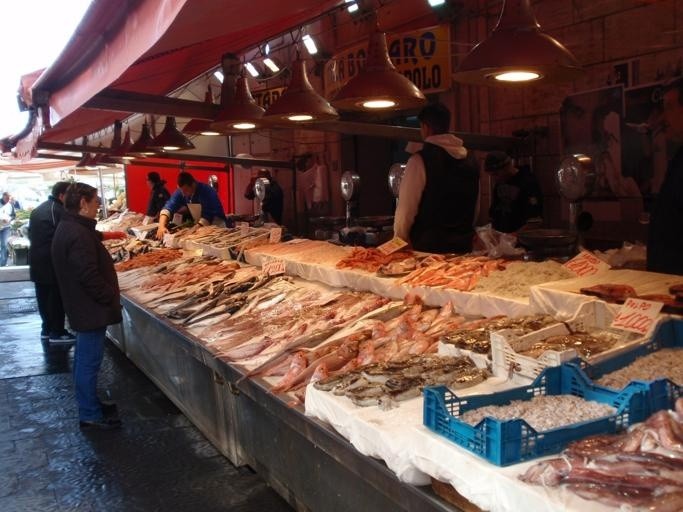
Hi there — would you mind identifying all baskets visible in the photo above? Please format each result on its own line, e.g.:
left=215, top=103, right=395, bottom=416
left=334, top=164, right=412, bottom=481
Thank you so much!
left=566, top=316, right=683, bottom=415
left=423, top=365, right=648, bottom=466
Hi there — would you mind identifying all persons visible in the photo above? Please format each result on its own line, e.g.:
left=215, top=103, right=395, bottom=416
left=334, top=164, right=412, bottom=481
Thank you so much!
left=482, top=151, right=544, bottom=245
left=27, top=180, right=128, bottom=345
left=155, top=170, right=228, bottom=243
left=393, top=101, right=483, bottom=258
left=567, top=148, right=645, bottom=259
left=51, top=181, right=125, bottom=431
left=573, top=104, right=619, bottom=162
left=244, top=167, right=286, bottom=228
left=645, top=77, right=681, bottom=276
left=9, top=197, right=23, bottom=211
left=0, top=189, right=19, bottom=267
left=141, top=170, right=172, bottom=226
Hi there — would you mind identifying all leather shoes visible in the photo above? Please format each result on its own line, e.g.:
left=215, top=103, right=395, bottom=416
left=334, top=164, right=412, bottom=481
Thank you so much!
left=98, top=400, right=117, bottom=413
left=79, top=416, right=120, bottom=431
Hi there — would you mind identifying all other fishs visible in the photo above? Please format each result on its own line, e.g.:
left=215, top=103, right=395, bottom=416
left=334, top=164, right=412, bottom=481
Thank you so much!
left=204, top=291, right=506, bottom=406
left=141, top=255, right=289, bottom=328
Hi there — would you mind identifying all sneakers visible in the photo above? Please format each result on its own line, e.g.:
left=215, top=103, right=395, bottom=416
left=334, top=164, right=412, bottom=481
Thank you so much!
left=41, top=330, right=49, bottom=339
left=49, top=332, right=76, bottom=342
